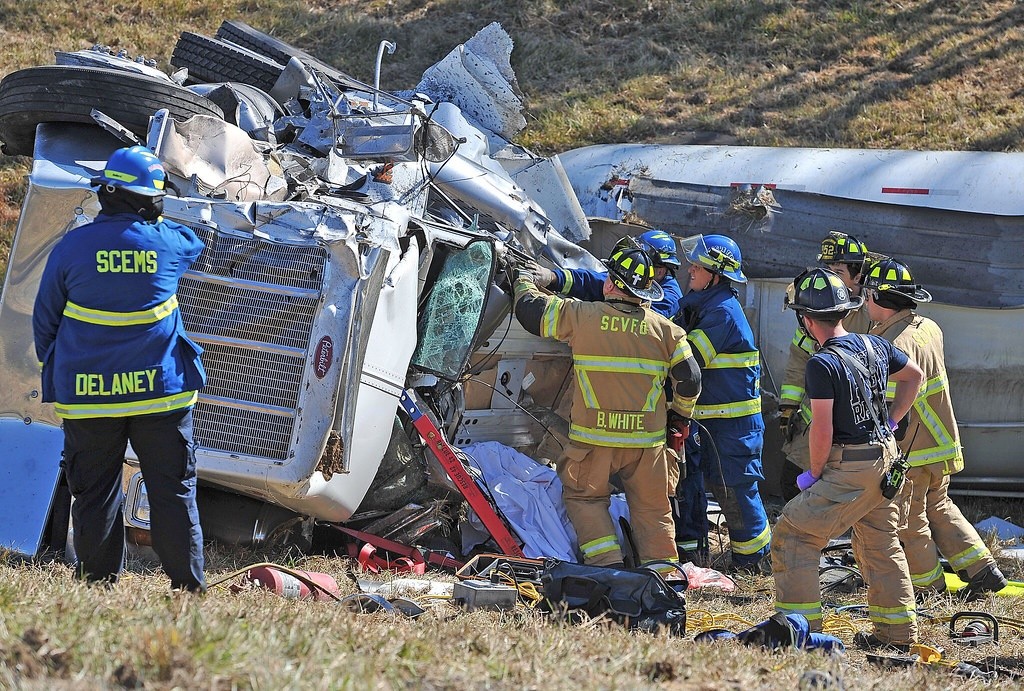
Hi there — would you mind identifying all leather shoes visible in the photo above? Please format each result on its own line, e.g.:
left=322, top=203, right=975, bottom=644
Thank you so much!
left=852, top=629, right=909, bottom=654
left=956, top=567, right=1008, bottom=602
left=914, top=584, right=948, bottom=605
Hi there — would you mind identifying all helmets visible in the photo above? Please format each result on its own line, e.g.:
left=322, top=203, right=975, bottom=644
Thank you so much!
left=687, top=235, right=748, bottom=284
left=857, top=259, right=933, bottom=303
left=94, top=145, right=167, bottom=197
left=635, top=229, right=681, bottom=265
left=787, top=267, right=864, bottom=314
left=820, top=232, right=868, bottom=263
left=603, top=245, right=665, bottom=302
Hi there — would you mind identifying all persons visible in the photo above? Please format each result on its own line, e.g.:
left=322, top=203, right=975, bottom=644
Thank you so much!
left=33, top=146, right=206, bottom=593
left=525, top=231, right=710, bottom=569
left=515, top=246, right=702, bottom=583
left=771, top=267, right=923, bottom=652
left=776, top=231, right=879, bottom=501
left=861, top=258, right=1007, bottom=602
left=663, top=233, right=773, bottom=575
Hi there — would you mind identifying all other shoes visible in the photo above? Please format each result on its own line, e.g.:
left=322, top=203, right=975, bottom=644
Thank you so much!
left=725, top=561, right=759, bottom=574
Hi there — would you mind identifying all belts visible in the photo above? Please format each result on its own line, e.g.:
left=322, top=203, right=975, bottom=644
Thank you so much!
left=840, top=448, right=884, bottom=461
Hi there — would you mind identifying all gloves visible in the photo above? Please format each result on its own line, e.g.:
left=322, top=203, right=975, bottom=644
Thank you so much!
left=779, top=406, right=803, bottom=443
left=524, top=259, right=553, bottom=286
left=512, top=268, right=535, bottom=283
left=888, top=417, right=899, bottom=433
left=796, top=472, right=817, bottom=492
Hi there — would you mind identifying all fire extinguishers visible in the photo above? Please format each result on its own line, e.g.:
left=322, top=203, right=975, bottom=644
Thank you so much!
left=207, top=551, right=340, bottom=603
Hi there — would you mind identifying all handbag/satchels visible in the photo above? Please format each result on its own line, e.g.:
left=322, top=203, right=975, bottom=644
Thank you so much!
left=536, top=558, right=689, bottom=640
left=697, top=612, right=844, bottom=655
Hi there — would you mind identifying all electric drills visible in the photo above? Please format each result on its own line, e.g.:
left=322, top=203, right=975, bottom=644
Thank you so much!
left=667, top=416, right=692, bottom=483
